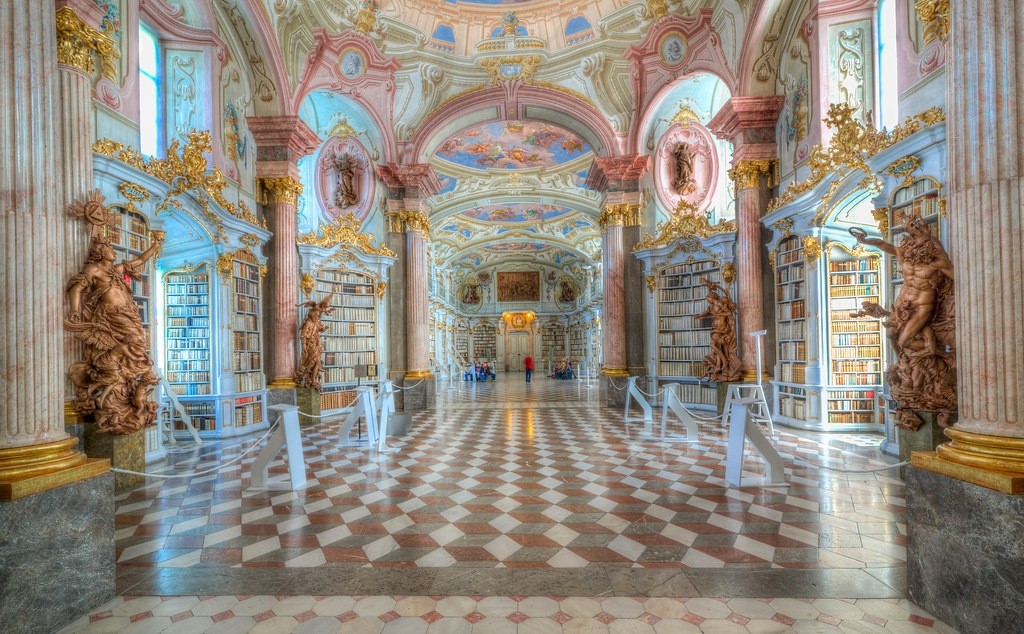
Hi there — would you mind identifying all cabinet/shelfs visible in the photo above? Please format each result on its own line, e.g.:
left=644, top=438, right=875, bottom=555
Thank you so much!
left=541, top=317, right=597, bottom=372
left=888, top=174, right=943, bottom=450
left=771, top=233, right=810, bottom=425
left=160, top=257, right=213, bottom=397
left=314, top=267, right=382, bottom=385
left=104, top=201, right=154, bottom=425
left=427, top=296, right=499, bottom=379
left=825, top=242, right=883, bottom=433
left=230, top=249, right=263, bottom=392
left=656, top=258, right=722, bottom=408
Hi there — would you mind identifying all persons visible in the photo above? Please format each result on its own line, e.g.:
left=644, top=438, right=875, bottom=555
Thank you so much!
left=465, top=360, right=497, bottom=382
left=524, top=355, right=534, bottom=382
left=333, top=159, right=354, bottom=197
left=857, top=216, right=954, bottom=389
left=294, top=289, right=336, bottom=391
left=694, top=278, right=742, bottom=382
left=553, top=358, right=578, bottom=378
left=68, top=237, right=160, bottom=419
left=674, top=142, right=694, bottom=182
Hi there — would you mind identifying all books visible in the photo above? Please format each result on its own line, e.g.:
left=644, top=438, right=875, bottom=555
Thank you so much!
left=657, top=261, right=721, bottom=405
left=304, top=270, right=377, bottom=411
left=776, top=177, right=939, bottom=424
left=102, top=211, right=262, bottom=430
left=429, top=318, right=587, bottom=370
